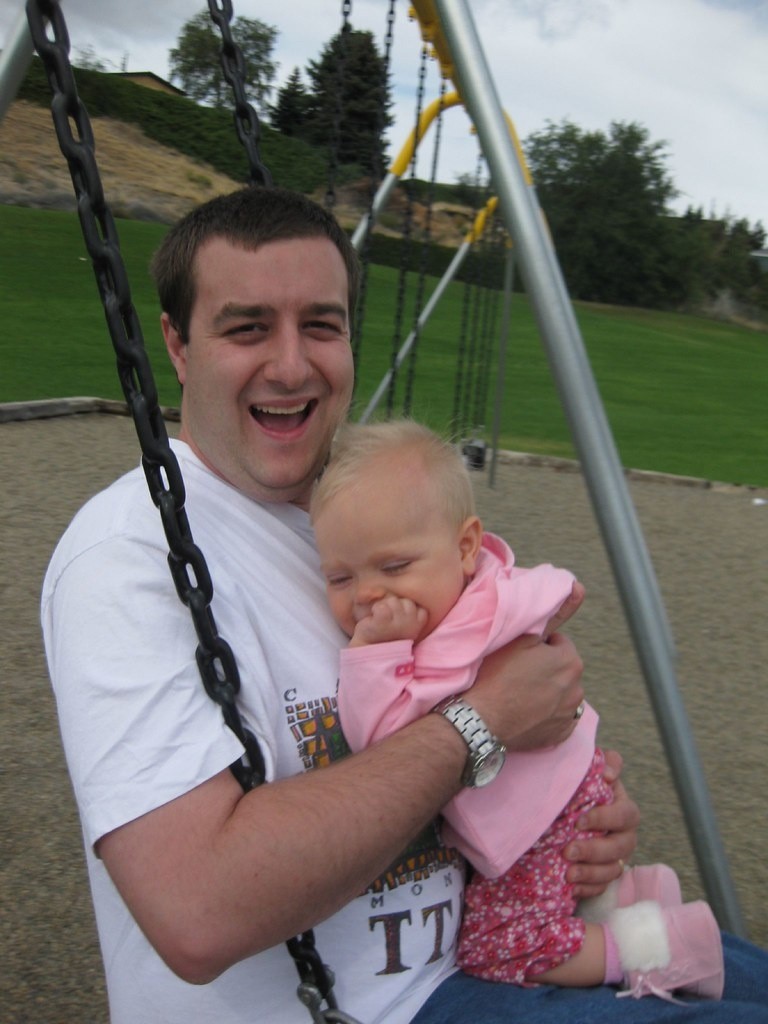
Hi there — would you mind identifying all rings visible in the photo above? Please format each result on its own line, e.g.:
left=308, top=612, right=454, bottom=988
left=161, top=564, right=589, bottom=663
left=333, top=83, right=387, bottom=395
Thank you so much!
left=575, top=705, right=585, bottom=718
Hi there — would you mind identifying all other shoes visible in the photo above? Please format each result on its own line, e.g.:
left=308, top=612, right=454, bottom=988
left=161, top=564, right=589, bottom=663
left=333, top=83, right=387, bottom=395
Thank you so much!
left=615, top=864, right=682, bottom=911
left=608, top=900, right=724, bottom=1006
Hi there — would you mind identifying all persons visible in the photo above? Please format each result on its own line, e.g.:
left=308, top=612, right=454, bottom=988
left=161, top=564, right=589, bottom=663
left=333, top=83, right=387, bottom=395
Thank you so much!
left=41, top=188, right=768, bottom=1024
left=307, top=419, right=723, bottom=997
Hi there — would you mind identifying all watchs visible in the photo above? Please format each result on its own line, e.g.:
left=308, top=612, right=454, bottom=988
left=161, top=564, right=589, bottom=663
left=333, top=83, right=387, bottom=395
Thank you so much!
left=432, top=694, right=506, bottom=787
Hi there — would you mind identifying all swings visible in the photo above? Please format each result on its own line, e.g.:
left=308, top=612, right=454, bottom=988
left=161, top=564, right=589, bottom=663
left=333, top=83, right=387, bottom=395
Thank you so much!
left=24, top=1, right=524, bottom=1024
